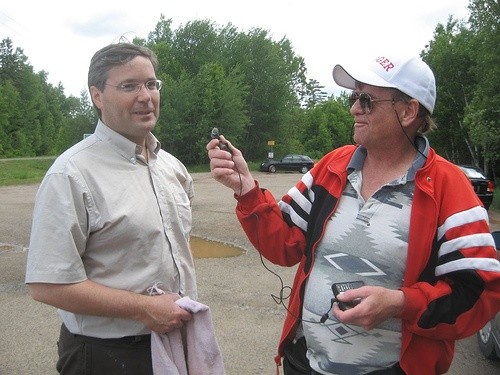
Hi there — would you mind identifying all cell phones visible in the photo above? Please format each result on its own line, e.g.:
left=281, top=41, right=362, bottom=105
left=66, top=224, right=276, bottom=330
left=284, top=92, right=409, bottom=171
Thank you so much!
left=332, top=281, right=365, bottom=311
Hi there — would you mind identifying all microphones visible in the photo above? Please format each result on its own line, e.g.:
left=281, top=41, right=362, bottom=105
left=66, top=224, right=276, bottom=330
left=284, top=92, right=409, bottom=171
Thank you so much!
left=211, top=128, right=227, bottom=151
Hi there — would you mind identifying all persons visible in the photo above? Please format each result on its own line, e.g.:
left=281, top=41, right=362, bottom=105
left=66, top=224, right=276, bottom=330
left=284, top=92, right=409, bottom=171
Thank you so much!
left=24, top=43, right=195, bottom=375
left=206, top=52, right=499, bottom=375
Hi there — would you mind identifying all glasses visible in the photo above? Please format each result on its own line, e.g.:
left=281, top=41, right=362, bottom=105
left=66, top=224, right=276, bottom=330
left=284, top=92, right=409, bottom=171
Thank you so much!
left=104, top=80, right=162, bottom=95
left=349, top=91, right=408, bottom=114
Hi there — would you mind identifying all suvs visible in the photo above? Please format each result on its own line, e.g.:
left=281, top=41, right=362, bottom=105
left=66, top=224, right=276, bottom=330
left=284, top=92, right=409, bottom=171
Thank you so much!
left=261, top=154, right=314, bottom=174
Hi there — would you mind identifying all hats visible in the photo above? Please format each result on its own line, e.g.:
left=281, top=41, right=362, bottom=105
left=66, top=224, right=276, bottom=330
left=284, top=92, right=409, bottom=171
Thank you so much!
left=332, top=55, right=436, bottom=115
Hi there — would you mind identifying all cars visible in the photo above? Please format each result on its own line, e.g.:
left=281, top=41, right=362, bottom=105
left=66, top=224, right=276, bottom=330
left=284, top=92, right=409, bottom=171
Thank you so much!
left=455, top=164, right=494, bottom=210
